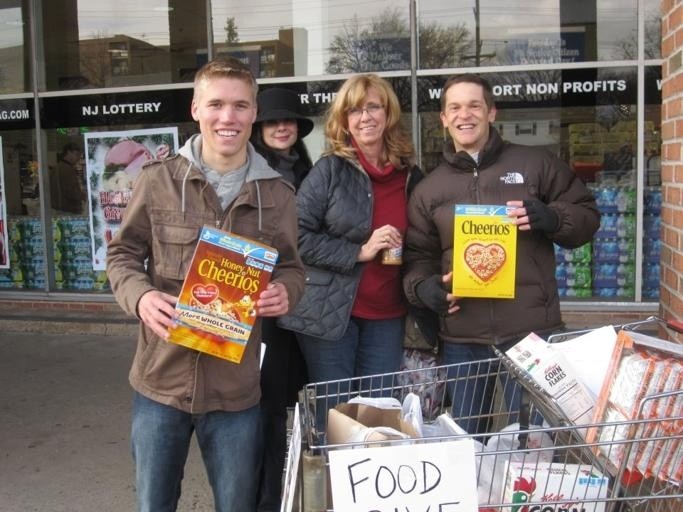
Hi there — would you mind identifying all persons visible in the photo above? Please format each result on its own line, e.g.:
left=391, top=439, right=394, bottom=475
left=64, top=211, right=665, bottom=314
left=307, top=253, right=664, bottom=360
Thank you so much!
left=276, top=68, right=424, bottom=416
left=397, top=74, right=601, bottom=446
left=102, top=54, right=306, bottom=512
left=245, top=87, right=315, bottom=480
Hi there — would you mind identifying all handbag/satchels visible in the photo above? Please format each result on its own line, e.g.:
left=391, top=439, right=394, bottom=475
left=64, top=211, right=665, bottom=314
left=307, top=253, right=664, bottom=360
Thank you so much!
left=396, top=308, right=448, bottom=421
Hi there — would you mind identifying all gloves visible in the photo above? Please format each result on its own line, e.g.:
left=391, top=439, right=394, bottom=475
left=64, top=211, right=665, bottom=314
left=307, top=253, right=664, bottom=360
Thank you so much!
left=415, top=273, right=450, bottom=318
left=522, top=198, right=559, bottom=232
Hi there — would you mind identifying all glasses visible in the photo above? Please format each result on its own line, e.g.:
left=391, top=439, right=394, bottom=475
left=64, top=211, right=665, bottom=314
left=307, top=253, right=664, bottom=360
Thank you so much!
left=347, top=103, right=387, bottom=115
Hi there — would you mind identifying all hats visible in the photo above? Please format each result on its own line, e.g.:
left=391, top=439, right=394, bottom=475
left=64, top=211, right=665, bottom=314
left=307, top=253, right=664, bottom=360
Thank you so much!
left=251, top=88, right=314, bottom=138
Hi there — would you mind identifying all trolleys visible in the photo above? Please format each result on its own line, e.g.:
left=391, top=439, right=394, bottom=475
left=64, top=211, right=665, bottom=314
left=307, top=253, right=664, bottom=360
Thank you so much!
left=280, top=315, right=683, bottom=512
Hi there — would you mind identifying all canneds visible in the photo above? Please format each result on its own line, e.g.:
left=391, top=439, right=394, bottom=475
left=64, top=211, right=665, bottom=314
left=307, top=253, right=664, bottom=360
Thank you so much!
left=381, top=234, right=403, bottom=265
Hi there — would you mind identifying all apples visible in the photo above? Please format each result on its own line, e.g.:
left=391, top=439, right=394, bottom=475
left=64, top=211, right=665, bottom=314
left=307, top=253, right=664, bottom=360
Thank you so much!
left=511, top=492, right=527, bottom=512
